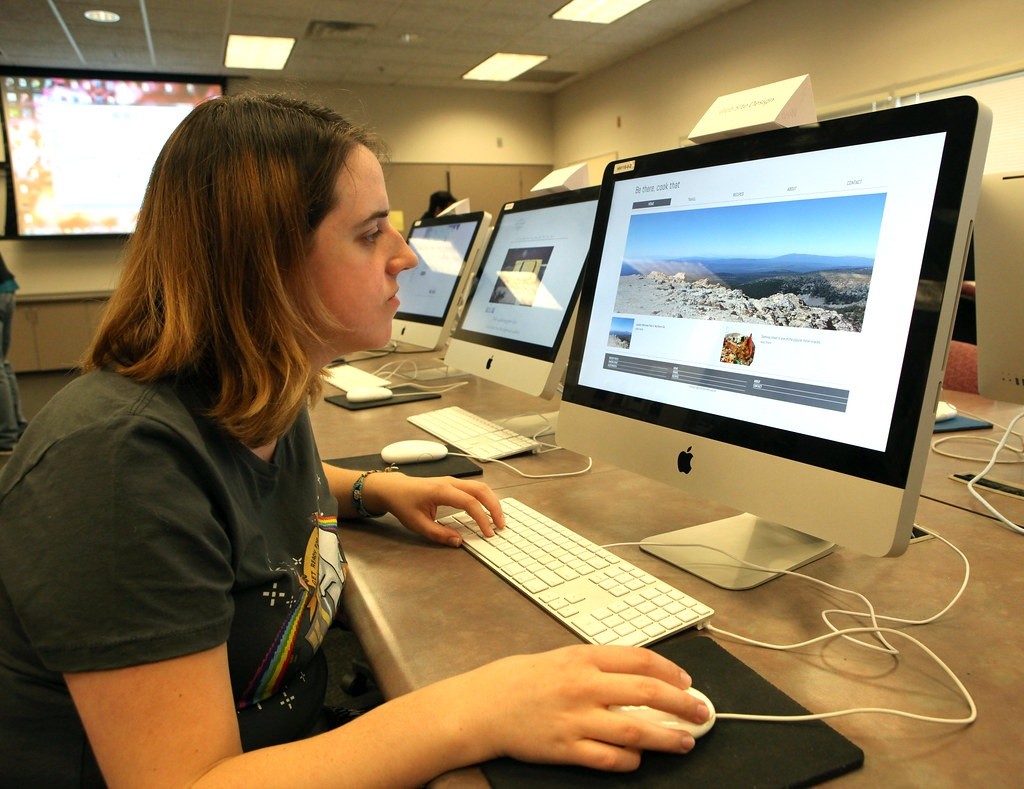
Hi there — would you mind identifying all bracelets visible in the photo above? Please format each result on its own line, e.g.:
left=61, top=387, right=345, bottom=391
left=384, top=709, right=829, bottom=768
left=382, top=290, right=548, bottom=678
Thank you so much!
left=353, top=463, right=399, bottom=518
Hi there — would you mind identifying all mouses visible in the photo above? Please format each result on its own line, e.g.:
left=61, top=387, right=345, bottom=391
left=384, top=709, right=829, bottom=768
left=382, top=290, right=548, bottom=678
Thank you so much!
left=381, top=440, right=448, bottom=464
left=346, top=388, right=393, bottom=402
left=935, top=400, right=958, bottom=422
left=607, top=685, right=716, bottom=738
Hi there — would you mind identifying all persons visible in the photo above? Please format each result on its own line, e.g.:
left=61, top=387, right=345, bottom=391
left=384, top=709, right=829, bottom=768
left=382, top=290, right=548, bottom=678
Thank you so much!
left=418, top=190, right=459, bottom=219
left=0, top=250, right=28, bottom=456
left=0, top=89, right=708, bottom=789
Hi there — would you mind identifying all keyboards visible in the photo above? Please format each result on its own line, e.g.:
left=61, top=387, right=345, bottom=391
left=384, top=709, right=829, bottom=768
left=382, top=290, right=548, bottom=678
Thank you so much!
left=430, top=497, right=714, bottom=649
left=318, top=364, right=392, bottom=392
left=407, top=406, right=541, bottom=462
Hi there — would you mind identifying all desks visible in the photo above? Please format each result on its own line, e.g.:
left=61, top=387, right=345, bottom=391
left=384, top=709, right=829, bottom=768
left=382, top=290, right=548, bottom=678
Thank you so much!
left=309, top=314, right=1024, bottom=789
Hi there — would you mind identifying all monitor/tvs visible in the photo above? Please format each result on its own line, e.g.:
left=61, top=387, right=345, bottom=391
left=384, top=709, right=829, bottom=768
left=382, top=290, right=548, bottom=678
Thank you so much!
left=394, top=94, right=1024, bottom=591
